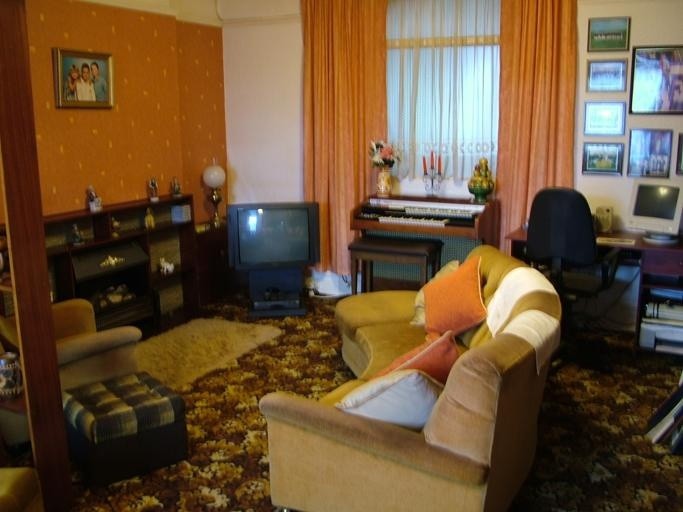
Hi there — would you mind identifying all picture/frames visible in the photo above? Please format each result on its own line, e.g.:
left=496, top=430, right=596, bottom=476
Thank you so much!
left=584, top=101, right=625, bottom=136
left=628, top=129, right=672, bottom=178
left=585, top=60, right=627, bottom=93
left=53, top=48, right=114, bottom=109
left=588, top=16, right=631, bottom=51
left=675, top=134, right=683, bottom=175
left=582, top=143, right=624, bottom=175
left=629, top=47, right=683, bottom=114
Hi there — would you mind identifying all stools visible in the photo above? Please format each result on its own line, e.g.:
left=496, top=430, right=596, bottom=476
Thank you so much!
left=62, top=371, right=188, bottom=487
left=348, top=236, right=445, bottom=295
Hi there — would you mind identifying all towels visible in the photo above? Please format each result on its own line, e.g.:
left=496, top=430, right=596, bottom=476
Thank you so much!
left=486, top=267, right=559, bottom=339
left=497, top=310, right=560, bottom=375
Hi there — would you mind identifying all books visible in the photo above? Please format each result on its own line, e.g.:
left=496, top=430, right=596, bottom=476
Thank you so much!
left=643, top=286, right=683, bottom=326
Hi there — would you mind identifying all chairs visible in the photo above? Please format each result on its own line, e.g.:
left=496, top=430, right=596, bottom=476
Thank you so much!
left=527, top=186, right=623, bottom=301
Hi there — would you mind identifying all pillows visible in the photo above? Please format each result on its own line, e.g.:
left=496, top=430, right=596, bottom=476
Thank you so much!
left=335, top=370, right=445, bottom=428
left=366, top=330, right=460, bottom=379
left=420, top=257, right=486, bottom=334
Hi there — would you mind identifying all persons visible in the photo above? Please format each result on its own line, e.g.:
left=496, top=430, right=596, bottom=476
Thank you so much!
left=89, top=63, right=107, bottom=100
left=64, top=64, right=81, bottom=101
left=75, top=63, right=96, bottom=101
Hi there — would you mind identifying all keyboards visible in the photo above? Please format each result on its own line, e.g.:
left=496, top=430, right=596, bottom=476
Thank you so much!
left=595, top=238, right=636, bottom=246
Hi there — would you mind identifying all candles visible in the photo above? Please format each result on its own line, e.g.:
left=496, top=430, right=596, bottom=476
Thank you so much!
left=422, top=155, right=427, bottom=175
left=430, top=150, right=434, bottom=169
left=438, top=155, right=442, bottom=175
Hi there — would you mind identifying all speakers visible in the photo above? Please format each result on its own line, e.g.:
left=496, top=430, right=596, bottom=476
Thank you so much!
left=595, top=206, right=612, bottom=234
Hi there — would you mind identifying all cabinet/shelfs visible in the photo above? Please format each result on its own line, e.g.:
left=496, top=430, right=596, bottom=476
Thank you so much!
left=0, top=191, right=204, bottom=339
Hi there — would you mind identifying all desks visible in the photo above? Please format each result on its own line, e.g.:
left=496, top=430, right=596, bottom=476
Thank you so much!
left=506, top=223, right=683, bottom=358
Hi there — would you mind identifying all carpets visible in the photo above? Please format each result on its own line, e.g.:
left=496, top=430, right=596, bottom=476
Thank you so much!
left=0, top=286, right=681, bottom=512
left=133, top=318, right=281, bottom=390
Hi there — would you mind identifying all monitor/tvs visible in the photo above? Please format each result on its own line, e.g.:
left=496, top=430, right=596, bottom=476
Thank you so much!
left=627, top=178, right=681, bottom=245
left=227, top=202, right=321, bottom=268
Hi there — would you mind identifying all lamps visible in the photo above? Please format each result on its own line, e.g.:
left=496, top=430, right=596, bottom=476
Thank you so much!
left=202, top=157, right=226, bottom=233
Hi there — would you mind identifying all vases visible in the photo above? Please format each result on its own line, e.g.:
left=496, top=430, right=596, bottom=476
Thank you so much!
left=376, top=167, right=391, bottom=198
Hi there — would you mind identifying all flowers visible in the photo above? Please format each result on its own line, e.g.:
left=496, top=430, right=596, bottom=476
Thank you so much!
left=367, top=138, right=401, bottom=167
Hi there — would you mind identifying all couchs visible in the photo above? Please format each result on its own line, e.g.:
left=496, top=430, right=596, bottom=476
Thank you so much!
left=0, top=298, right=142, bottom=454
left=259, top=245, right=561, bottom=512
